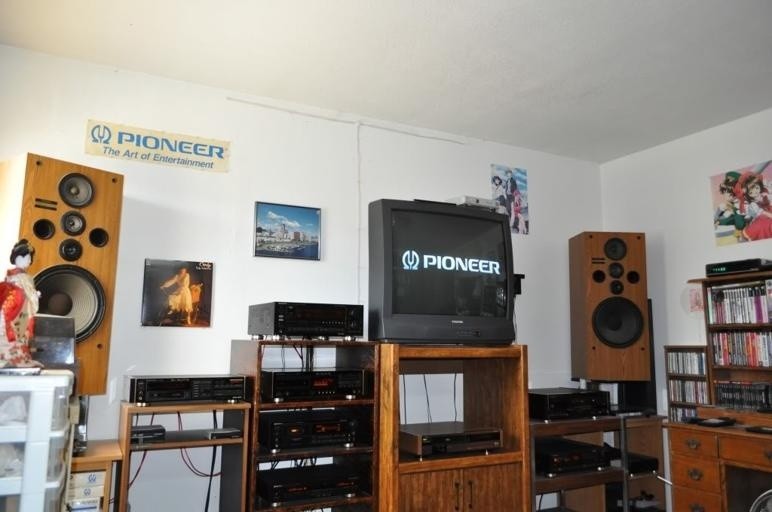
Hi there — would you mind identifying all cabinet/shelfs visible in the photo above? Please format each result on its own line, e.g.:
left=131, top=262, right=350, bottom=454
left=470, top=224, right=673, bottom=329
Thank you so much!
left=697, top=269, right=771, bottom=428
left=0, top=370, right=72, bottom=512
left=378, top=341, right=532, bottom=512
left=119, top=399, right=250, bottom=511
left=662, top=341, right=716, bottom=425
left=231, top=339, right=377, bottom=511
left=65, top=439, right=123, bottom=511
left=531, top=417, right=669, bottom=512
left=668, top=413, right=771, bottom=512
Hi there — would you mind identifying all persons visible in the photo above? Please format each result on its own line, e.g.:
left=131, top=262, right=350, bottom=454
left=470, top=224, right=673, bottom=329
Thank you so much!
left=734, top=171, right=772, bottom=239
left=159, top=267, right=193, bottom=325
left=492, top=175, right=507, bottom=207
left=0, top=237, right=45, bottom=368
left=509, top=190, right=528, bottom=234
left=714, top=171, right=753, bottom=239
left=495, top=195, right=509, bottom=216
left=504, top=170, right=517, bottom=212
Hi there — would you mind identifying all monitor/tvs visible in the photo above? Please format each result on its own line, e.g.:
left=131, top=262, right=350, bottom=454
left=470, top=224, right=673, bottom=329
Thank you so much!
left=368, top=199, right=517, bottom=346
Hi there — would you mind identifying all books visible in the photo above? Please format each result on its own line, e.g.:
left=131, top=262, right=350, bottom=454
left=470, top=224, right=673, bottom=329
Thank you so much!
left=706, top=279, right=772, bottom=325
left=668, top=379, right=710, bottom=404
left=666, top=352, right=706, bottom=376
left=710, top=331, right=772, bottom=367
left=670, top=407, right=695, bottom=422
left=713, top=379, right=771, bottom=412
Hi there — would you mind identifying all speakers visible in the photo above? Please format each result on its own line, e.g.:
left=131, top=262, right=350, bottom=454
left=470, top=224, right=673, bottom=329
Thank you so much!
left=18, top=151, right=124, bottom=397
left=568, top=231, right=651, bottom=382
